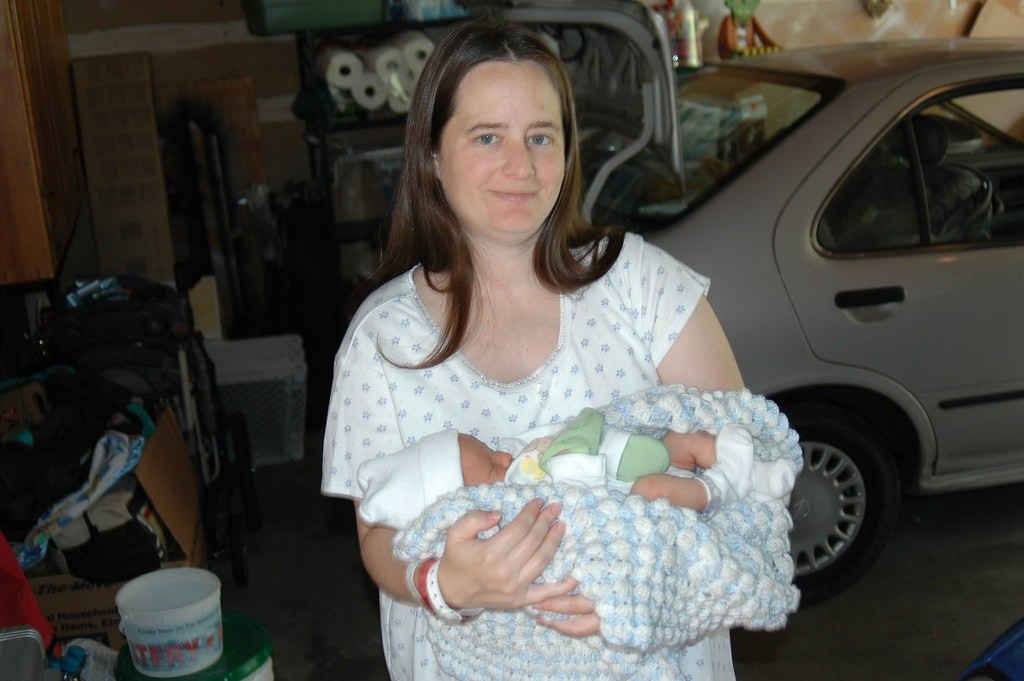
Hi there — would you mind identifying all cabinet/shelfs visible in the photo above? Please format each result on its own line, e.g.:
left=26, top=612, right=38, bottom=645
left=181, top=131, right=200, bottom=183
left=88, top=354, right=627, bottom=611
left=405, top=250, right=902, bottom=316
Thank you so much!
left=296, top=18, right=472, bottom=301
left=0, top=0, right=85, bottom=285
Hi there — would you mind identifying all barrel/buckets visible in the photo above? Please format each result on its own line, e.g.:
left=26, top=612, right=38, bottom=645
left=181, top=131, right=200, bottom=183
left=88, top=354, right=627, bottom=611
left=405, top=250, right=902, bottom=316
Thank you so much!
left=116, top=568, right=222, bottom=677
left=114, top=613, right=274, bottom=681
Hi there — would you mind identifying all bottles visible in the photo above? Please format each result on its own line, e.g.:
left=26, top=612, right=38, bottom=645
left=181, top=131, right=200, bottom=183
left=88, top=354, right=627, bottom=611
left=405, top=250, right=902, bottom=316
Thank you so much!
left=47, top=641, right=85, bottom=681
left=652, top=0, right=699, bottom=69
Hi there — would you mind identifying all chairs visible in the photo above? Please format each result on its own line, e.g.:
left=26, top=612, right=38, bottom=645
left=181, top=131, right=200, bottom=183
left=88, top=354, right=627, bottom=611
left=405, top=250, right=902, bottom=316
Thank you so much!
left=886, top=115, right=992, bottom=242
left=30, top=274, right=261, bottom=586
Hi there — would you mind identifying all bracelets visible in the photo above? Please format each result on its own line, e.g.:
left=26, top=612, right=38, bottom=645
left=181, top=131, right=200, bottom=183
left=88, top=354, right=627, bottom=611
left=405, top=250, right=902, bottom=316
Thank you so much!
left=406, top=557, right=485, bottom=624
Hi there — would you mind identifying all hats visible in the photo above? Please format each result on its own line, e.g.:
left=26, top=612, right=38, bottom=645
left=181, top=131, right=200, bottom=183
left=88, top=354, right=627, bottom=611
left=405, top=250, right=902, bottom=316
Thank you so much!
left=356, top=428, right=466, bottom=529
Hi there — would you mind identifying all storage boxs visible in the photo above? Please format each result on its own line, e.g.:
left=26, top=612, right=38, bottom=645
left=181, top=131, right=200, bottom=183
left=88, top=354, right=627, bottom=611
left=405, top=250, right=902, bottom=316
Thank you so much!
left=27, top=403, right=209, bottom=649
left=239, top=0, right=385, bottom=37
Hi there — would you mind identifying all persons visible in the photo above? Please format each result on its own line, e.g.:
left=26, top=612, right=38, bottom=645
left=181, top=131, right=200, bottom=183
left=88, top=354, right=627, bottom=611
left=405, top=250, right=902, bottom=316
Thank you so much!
left=357, top=408, right=797, bottom=529
left=322, top=19, right=746, bottom=681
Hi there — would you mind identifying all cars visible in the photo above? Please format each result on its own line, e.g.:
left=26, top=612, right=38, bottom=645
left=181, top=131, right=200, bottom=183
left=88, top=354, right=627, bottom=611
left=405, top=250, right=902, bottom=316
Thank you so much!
left=445, top=0, right=1024, bottom=610
